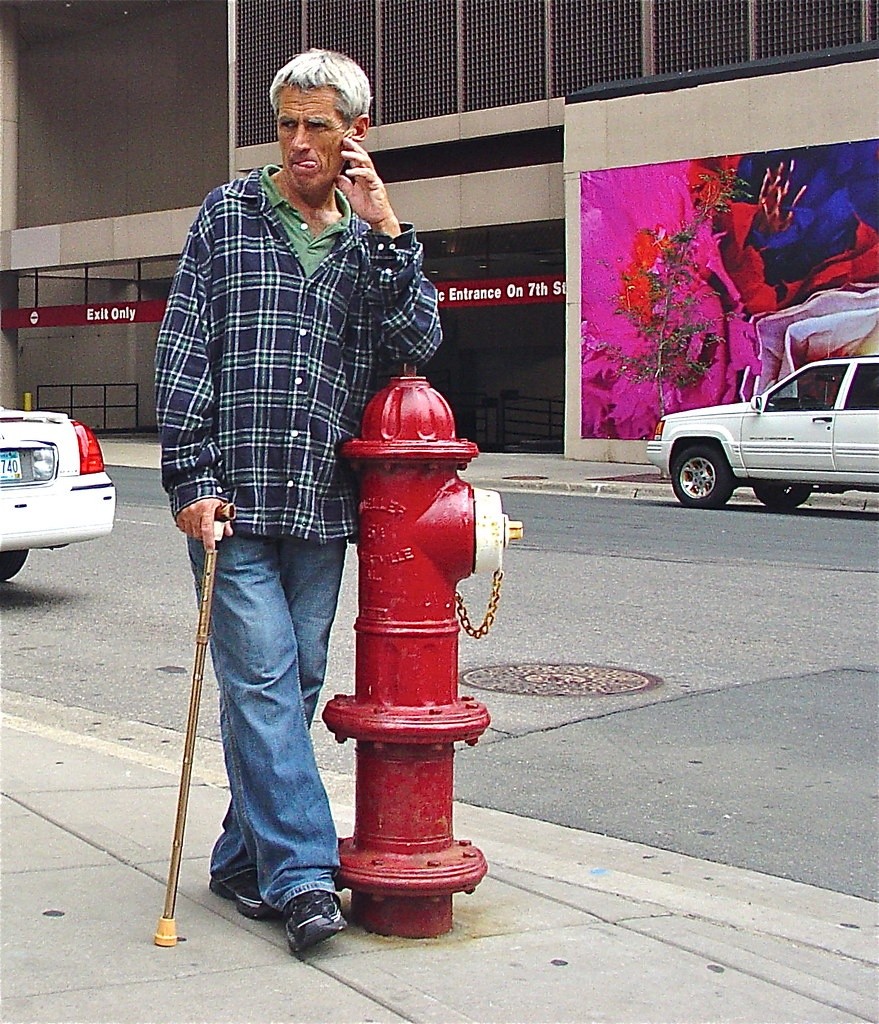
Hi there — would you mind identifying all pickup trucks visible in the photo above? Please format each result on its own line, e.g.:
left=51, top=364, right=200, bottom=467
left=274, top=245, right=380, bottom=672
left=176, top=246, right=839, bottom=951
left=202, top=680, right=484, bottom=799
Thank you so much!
left=646, top=356, right=879, bottom=512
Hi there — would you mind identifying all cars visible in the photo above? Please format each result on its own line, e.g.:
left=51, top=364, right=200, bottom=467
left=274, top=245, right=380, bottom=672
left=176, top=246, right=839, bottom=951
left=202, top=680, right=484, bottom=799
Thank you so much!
left=0, top=401, right=117, bottom=582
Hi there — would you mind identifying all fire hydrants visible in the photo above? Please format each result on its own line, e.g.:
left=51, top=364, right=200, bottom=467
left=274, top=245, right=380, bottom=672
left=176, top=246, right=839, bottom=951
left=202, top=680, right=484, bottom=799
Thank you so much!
left=318, top=355, right=525, bottom=946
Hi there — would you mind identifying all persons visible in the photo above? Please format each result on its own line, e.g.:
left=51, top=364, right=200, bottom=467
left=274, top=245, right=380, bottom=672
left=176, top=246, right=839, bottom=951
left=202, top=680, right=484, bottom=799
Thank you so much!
left=153, top=49, right=444, bottom=948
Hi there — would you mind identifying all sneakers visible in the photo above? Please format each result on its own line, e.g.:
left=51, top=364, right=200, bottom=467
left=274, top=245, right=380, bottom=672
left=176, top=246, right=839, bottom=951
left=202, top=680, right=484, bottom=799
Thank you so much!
left=210, top=871, right=275, bottom=919
left=284, top=891, right=348, bottom=952
